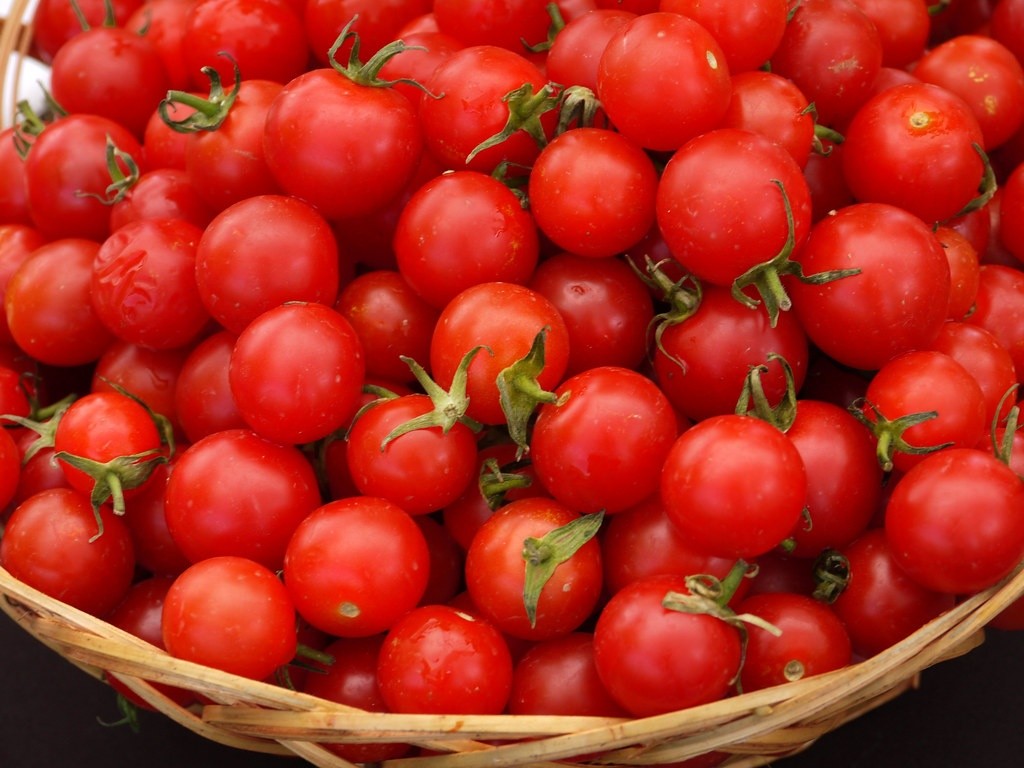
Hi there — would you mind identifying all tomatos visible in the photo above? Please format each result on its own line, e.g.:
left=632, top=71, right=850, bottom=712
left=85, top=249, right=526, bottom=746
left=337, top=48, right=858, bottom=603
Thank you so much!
left=0, top=0, right=1024, bottom=763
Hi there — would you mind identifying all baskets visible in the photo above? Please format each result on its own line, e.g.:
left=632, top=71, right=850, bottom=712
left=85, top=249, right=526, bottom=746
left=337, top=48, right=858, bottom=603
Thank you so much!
left=0, top=0, right=1024, bottom=768
